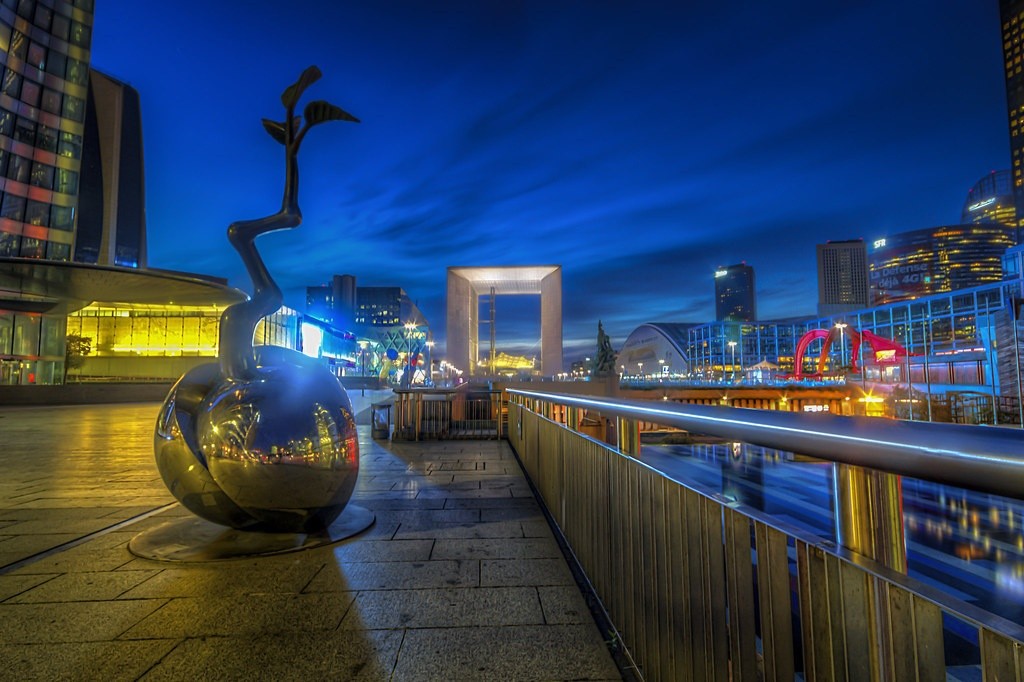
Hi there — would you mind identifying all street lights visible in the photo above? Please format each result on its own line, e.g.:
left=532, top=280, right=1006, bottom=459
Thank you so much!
left=357, top=341, right=369, bottom=397
left=638, top=362, right=643, bottom=378
left=426, top=341, right=434, bottom=385
left=441, top=360, right=463, bottom=385
left=659, top=359, right=665, bottom=377
left=729, top=341, right=737, bottom=376
left=405, top=322, right=416, bottom=389
